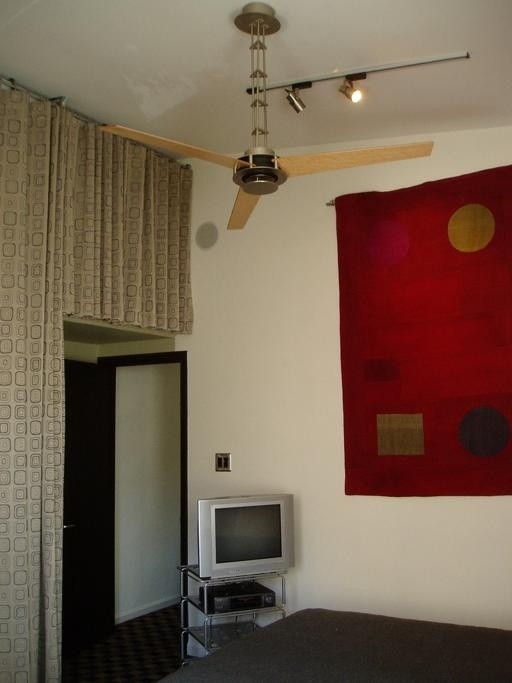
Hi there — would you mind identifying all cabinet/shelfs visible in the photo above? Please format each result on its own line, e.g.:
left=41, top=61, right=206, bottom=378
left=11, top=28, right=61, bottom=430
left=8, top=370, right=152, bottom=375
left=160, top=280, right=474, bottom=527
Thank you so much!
left=175, top=564, right=286, bottom=671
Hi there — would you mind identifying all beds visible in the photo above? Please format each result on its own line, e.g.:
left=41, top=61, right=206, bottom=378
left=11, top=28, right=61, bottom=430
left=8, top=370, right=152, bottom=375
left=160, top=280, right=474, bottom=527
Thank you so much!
left=154, top=606, right=510, bottom=683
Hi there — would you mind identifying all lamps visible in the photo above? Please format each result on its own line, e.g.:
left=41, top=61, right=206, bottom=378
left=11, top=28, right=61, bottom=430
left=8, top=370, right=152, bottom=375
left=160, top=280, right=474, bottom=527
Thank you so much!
left=276, top=72, right=373, bottom=115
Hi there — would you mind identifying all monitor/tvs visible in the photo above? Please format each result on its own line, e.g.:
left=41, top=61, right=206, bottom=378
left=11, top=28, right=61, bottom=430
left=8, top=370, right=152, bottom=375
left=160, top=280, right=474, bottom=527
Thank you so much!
left=198, top=493, right=295, bottom=581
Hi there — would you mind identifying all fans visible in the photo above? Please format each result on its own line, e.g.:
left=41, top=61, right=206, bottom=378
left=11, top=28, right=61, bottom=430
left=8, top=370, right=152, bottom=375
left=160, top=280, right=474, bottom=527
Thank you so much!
left=94, top=6, right=435, bottom=230
